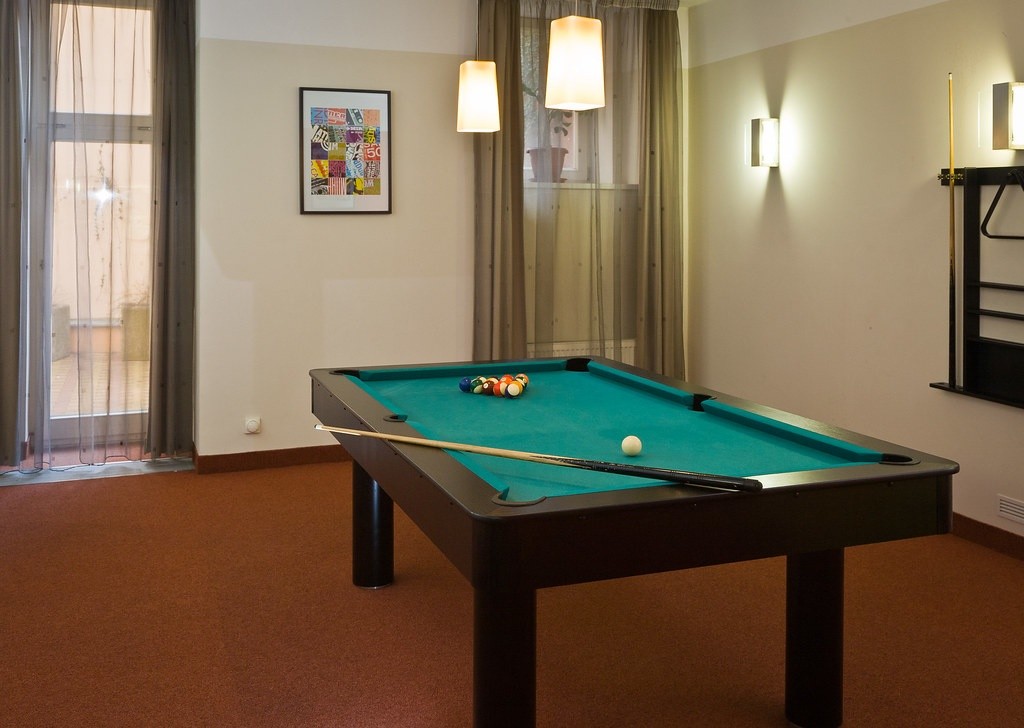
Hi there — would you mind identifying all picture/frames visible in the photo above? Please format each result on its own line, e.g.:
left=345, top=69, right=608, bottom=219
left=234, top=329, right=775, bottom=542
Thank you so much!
left=299, top=87, right=392, bottom=214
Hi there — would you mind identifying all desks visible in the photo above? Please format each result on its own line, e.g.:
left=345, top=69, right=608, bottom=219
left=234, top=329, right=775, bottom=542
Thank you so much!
left=309, top=354, right=962, bottom=728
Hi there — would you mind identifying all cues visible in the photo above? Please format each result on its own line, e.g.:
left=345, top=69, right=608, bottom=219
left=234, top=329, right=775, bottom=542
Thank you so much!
left=310, top=421, right=767, bottom=496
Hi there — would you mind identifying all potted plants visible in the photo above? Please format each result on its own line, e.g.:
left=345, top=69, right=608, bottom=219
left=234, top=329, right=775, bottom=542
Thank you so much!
left=523, top=67, right=573, bottom=183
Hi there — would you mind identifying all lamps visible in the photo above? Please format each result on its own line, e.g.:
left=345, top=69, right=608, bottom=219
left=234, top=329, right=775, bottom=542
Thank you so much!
left=992, top=82, right=1024, bottom=151
left=750, top=117, right=781, bottom=167
left=457, top=0, right=500, bottom=132
left=544, top=0, right=605, bottom=112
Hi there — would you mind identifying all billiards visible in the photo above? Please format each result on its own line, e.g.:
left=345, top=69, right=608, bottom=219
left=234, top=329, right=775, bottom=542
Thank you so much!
left=621, top=435, right=643, bottom=457
left=458, top=372, right=530, bottom=400
